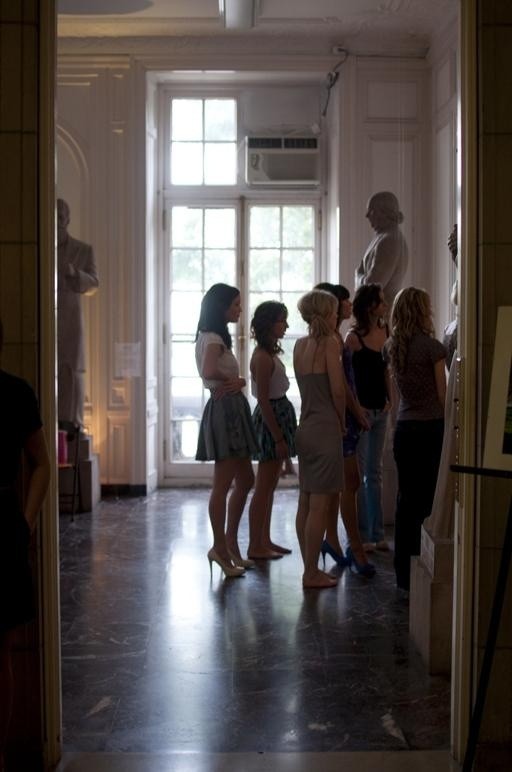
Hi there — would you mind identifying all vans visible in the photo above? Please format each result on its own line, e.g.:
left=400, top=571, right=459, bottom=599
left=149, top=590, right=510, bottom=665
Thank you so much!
left=208, top=539, right=389, bottom=587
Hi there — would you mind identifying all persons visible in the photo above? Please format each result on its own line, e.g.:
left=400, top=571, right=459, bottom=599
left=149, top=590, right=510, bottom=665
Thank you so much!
left=355, top=191, right=410, bottom=304
left=383, top=286, right=448, bottom=590
left=57, top=199, right=100, bottom=434
left=247, top=301, right=298, bottom=560
left=443, top=223, right=458, bottom=371
left=195, top=283, right=264, bottom=581
left=292, top=289, right=346, bottom=585
left=0, top=319, right=51, bottom=772
left=311, top=283, right=353, bottom=567
left=343, top=285, right=394, bottom=574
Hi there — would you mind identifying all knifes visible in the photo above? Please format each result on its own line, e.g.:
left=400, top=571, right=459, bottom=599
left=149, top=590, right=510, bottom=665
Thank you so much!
left=244, top=135, right=321, bottom=190
